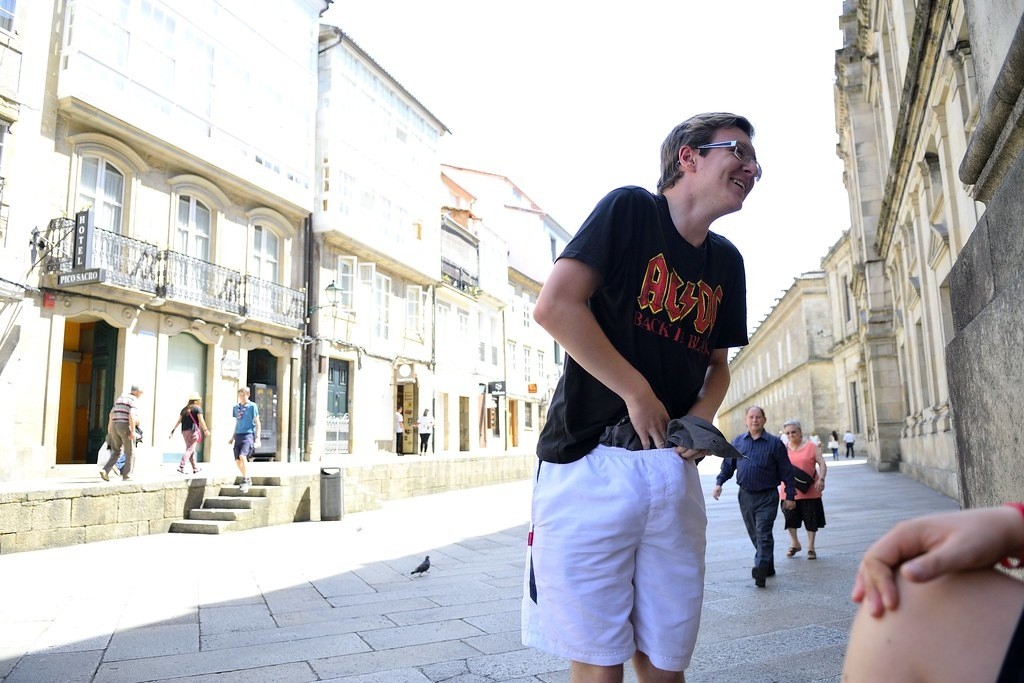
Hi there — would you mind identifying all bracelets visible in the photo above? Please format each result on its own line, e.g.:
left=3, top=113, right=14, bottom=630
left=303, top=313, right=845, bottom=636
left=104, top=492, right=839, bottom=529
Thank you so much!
left=203, top=429, right=207, bottom=431
left=999, top=501, right=1024, bottom=569
left=820, top=479, right=824, bottom=482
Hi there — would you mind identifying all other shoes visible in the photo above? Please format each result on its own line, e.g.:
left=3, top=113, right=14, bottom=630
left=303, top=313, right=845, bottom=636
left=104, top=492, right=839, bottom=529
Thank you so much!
left=177, top=468, right=187, bottom=474
left=752, top=567, right=766, bottom=587
left=113, top=465, right=120, bottom=476
left=100, top=469, right=109, bottom=481
left=239, top=478, right=252, bottom=493
left=193, top=468, right=201, bottom=473
left=123, top=475, right=133, bottom=481
left=752, top=566, right=776, bottom=576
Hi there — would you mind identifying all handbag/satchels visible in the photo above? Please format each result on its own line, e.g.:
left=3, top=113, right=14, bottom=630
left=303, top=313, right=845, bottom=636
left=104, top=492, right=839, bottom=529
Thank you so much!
left=196, top=428, right=201, bottom=443
left=792, top=466, right=814, bottom=494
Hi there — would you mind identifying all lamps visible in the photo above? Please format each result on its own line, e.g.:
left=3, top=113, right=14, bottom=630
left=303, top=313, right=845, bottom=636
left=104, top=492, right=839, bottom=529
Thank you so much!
left=233, top=316, right=247, bottom=325
left=149, top=296, right=166, bottom=306
left=308, top=280, right=344, bottom=313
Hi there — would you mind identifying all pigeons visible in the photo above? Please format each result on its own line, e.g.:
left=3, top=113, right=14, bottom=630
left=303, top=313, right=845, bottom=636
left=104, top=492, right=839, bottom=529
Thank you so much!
left=411, top=556, right=431, bottom=576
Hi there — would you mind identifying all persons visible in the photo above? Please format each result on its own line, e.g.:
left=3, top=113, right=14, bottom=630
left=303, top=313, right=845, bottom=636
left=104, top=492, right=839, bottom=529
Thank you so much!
left=809, top=432, right=820, bottom=446
left=228, top=386, right=262, bottom=493
left=169, top=391, right=210, bottom=474
left=779, top=420, right=828, bottom=560
left=417, top=408, right=435, bottom=456
left=98, top=384, right=144, bottom=481
left=712, top=406, right=796, bottom=588
left=521, top=112, right=763, bottom=683
left=838, top=506, right=1024, bottom=683
left=843, top=430, right=856, bottom=458
left=396, top=405, right=405, bottom=456
left=829, top=430, right=839, bottom=461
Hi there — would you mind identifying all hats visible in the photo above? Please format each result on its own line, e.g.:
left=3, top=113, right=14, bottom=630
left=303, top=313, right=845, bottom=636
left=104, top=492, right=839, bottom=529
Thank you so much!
left=188, top=392, right=202, bottom=400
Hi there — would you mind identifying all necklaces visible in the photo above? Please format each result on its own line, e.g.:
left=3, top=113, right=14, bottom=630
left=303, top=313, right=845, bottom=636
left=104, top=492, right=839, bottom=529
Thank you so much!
left=237, top=400, right=250, bottom=418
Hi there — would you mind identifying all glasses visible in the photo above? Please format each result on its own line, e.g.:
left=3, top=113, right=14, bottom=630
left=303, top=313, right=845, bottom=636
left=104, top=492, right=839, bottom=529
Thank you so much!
left=678, top=140, right=763, bottom=182
left=785, top=430, right=797, bottom=435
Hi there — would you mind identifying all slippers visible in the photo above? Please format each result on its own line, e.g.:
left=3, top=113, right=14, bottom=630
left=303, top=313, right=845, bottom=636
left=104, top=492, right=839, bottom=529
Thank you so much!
left=808, top=550, right=817, bottom=559
left=787, top=546, right=801, bottom=556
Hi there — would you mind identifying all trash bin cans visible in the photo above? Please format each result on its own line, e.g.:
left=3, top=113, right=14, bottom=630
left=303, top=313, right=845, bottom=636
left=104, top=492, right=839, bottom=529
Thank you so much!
left=319, top=467, right=343, bottom=521
